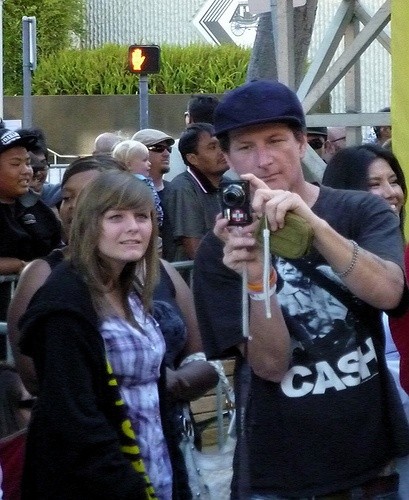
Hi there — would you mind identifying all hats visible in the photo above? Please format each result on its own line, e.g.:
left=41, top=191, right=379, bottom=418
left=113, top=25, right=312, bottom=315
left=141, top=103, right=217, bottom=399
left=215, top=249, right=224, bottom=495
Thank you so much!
left=131, top=129, right=175, bottom=146
left=0, top=128, right=40, bottom=152
left=210, top=80, right=306, bottom=136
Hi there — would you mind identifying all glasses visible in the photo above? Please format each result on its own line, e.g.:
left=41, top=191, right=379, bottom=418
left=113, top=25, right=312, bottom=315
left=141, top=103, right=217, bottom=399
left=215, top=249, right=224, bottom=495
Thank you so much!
left=148, top=145, right=172, bottom=153
left=36, top=160, right=52, bottom=171
left=306, top=138, right=327, bottom=150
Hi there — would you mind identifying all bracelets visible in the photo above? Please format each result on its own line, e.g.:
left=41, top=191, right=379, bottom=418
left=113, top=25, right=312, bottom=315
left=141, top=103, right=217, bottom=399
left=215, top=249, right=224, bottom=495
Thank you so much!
left=247, top=267, right=277, bottom=301
left=331, top=240, right=359, bottom=276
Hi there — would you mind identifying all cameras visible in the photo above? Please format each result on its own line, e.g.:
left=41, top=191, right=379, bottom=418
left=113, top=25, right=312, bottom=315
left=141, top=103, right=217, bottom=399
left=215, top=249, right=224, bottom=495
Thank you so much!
left=219, top=179, right=253, bottom=226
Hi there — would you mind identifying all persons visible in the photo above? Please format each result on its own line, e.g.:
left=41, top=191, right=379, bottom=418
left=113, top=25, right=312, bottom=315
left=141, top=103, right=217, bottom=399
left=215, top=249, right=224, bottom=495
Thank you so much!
left=0, top=117, right=230, bottom=437
left=321, top=145, right=409, bottom=500
left=193, top=79, right=408, bottom=500
left=7, top=154, right=219, bottom=451
left=275, top=255, right=347, bottom=340
left=17, top=168, right=194, bottom=500
left=304, top=106, right=392, bottom=165
